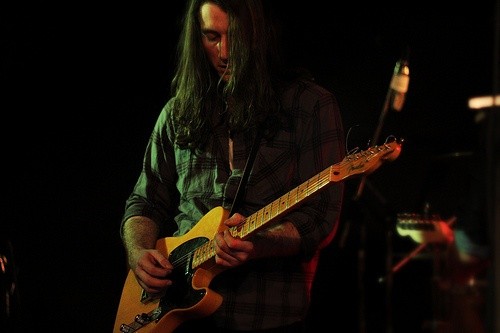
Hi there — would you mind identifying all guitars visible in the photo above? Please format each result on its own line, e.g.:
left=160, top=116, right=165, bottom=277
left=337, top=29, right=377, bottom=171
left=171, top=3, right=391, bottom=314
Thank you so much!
left=397, top=212, right=491, bottom=265
left=111, top=140, right=402, bottom=333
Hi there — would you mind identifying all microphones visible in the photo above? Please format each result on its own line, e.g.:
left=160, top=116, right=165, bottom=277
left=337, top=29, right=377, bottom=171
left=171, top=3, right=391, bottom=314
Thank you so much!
left=359, top=60, right=410, bottom=203
left=0, top=255, right=16, bottom=296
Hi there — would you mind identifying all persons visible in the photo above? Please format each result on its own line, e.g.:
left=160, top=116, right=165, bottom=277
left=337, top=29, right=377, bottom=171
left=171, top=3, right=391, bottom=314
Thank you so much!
left=119, top=0, right=344, bottom=333
